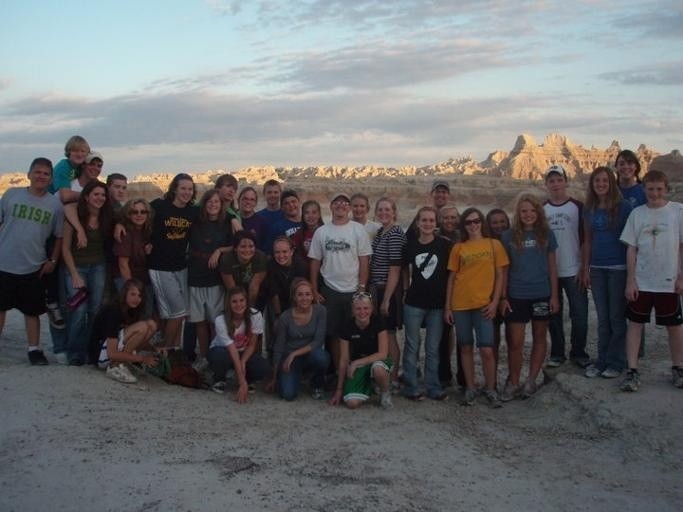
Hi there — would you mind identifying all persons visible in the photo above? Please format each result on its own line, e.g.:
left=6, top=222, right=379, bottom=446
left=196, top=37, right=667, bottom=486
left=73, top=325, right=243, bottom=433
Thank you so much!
left=48, top=135, right=648, bottom=413
left=618, top=170, right=682, bottom=394
left=0, top=155, right=67, bottom=367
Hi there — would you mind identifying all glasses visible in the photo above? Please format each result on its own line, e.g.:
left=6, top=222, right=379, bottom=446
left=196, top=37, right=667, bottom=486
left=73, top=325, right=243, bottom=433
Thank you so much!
left=332, top=201, right=349, bottom=206
left=463, top=218, right=481, bottom=226
left=129, top=209, right=147, bottom=216
left=351, top=292, right=372, bottom=299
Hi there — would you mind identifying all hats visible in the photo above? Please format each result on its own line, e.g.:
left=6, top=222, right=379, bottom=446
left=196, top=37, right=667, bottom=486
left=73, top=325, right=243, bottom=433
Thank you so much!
left=545, top=164, right=566, bottom=179
left=84, top=151, right=105, bottom=164
left=330, top=192, right=351, bottom=205
left=433, top=181, right=450, bottom=188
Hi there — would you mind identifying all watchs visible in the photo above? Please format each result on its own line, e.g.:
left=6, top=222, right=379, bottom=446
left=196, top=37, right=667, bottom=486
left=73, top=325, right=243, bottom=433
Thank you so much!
left=48, top=257, right=57, bottom=264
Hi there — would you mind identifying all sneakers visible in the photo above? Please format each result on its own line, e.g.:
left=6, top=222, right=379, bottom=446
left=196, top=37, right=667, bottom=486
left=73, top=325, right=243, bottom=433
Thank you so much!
left=105, top=363, right=138, bottom=383
left=46, top=303, right=66, bottom=330
left=381, top=394, right=392, bottom=410
left=27, top=349, right=49, bottom=365
left=55, top=353, right=70, bottom=366
left=195, top=360, right=326, bottom=400
left=672, top=366, right=682, bottom=387
left=408, top=359, right=640, bottom=408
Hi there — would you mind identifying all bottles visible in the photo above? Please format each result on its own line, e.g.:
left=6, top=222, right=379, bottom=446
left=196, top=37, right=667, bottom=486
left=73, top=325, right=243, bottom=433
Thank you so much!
left=67, top=285, right=90, bottom=310
